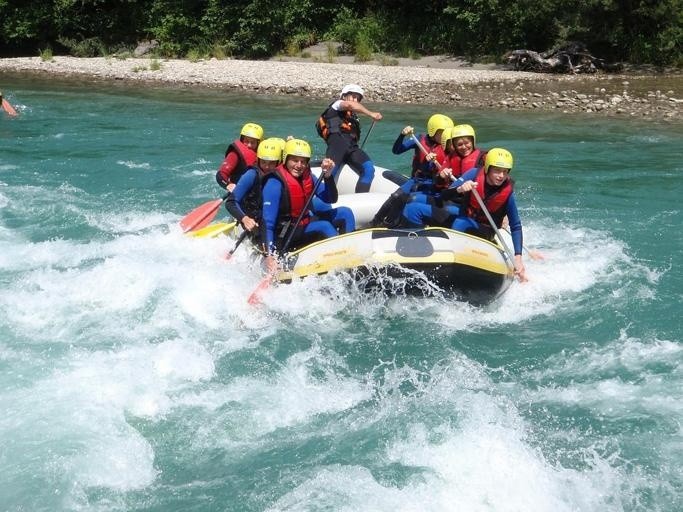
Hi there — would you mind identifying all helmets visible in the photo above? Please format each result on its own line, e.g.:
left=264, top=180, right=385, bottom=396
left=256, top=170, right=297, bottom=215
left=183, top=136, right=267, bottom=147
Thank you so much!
left=240, top=122, right=264, bottom=140
left=427, top=113, right=476, bottom=150
left=484, top=147, right=513, bottom=174
left=256, top=137, right=311, bottom=165
left=339, top=84, right=364, bottom=102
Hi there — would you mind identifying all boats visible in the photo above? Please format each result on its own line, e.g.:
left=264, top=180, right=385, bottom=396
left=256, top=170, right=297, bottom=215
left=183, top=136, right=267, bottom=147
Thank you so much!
left=228, top=159, right=518, bottom=307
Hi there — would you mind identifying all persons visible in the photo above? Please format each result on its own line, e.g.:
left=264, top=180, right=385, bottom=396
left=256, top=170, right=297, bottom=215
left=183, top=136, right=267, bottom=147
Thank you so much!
left=325, top=83, right=382, bottom=192
left=217, top=123, right=355, bottom=278
left=369, top=113, right=528, bottom=284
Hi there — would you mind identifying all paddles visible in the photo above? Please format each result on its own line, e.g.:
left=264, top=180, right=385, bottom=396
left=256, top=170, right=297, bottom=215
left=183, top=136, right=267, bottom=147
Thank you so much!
left=192, top=219, right=240, bottom=237
left=247, top=171, right=324, bottom=307
left=178, top=192, right=231, bottom=233
